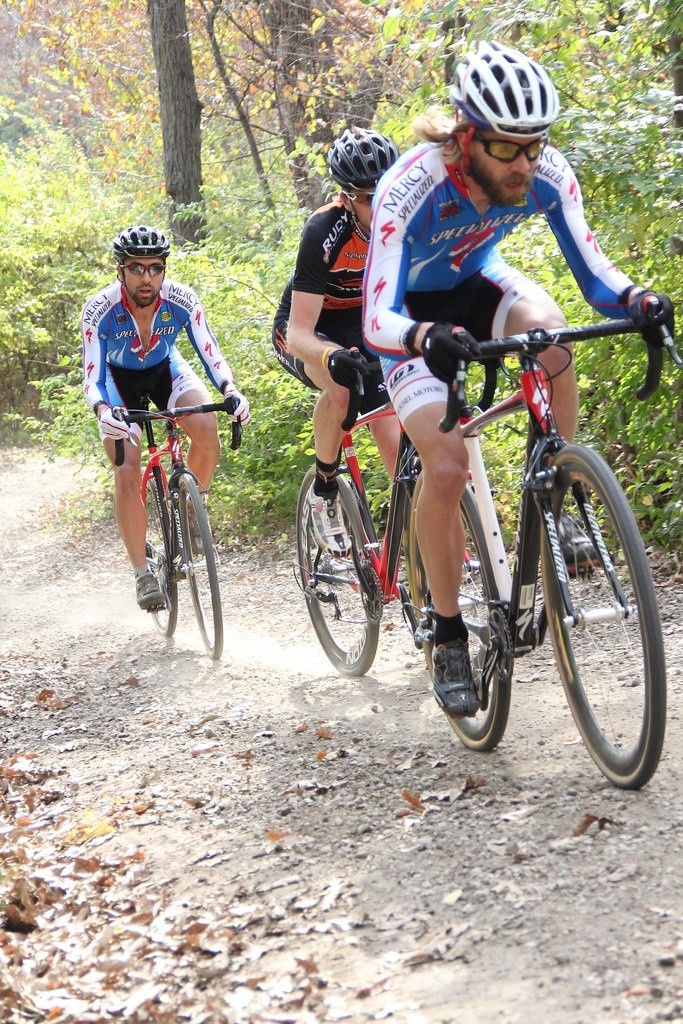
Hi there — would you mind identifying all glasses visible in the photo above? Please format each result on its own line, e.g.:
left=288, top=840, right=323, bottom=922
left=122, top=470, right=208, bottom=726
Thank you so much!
left=119, top=264, right=167, bottom=277
left=469, top=131, right=549, bottom=164
left=344, top=188, right=375, bottom=207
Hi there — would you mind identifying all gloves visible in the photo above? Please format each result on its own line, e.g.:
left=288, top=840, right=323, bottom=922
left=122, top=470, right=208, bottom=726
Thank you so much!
left=222, top=389, right=251, bottom=426
left=96, top=408, right=129, bottom=441
left=617, top=285, right=673, bottom=350
left=420, top=320, right=482, bottom=385
left=326, top=347, right=371, bottom=390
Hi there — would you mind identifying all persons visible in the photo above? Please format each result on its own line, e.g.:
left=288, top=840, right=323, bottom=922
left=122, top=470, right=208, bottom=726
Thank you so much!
left=81, top=225, right=250, bottom=610
left=361, top=38, right=676, bottom=718
left=271, top=127, right=400, bottom=559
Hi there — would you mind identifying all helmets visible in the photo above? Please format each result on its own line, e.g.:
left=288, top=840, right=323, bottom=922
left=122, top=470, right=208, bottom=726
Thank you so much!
left=328, top=126, right=400, bottom=191
left=449, top=39, right=559, bottom=139
left=113, top=225, right=171, bottom=264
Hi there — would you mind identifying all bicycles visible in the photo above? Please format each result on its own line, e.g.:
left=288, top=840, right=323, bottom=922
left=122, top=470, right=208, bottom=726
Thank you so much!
left=111, top=396, right=245, bottom=660
left=291, top=294, right=683, bottom=790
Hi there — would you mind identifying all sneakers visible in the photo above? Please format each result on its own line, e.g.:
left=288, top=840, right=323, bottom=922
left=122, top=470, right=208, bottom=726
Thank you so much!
left=548, top=506, right=604, bottom=572
left=185, top=494, right=212, bottom=556
left=134, top=572, right=164, bottom=609
left=303, top=479, right=353, bottom=558
left=430, top=626, right=480, bottom=719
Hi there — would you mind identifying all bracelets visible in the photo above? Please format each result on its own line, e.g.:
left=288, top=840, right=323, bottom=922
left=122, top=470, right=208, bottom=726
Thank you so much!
left=622, top=285, right=639, bottom=317
left=322, top=347, right=337, bottom=370
left=406, top=322, right=421, bottom=357
